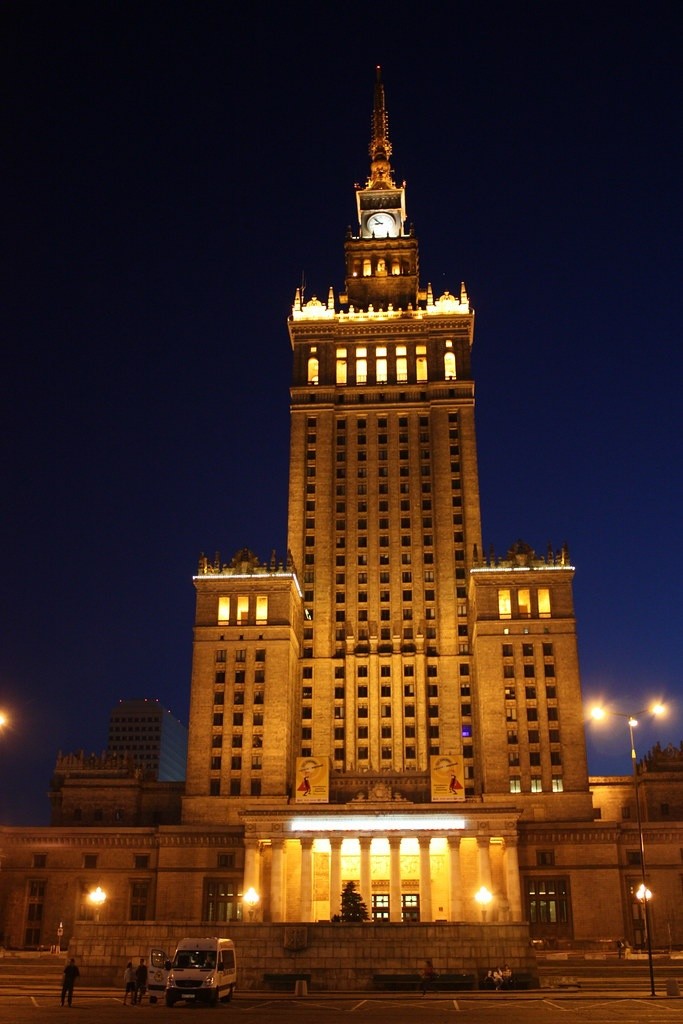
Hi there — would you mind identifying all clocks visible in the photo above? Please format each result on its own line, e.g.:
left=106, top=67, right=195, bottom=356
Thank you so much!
left=365, top=212, right=397, bottom=235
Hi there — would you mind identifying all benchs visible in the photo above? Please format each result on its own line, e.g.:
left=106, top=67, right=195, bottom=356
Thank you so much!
left=480, top=973, right=533, bottom=990
left=371, top=973, right=423, bottom=991
left=263, top=973, right=312, bottom=994
left=431, top=974, right=474, bottom=992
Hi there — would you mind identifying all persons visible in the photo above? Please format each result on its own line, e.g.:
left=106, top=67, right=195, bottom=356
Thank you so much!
left=123, top=962, right=136, bottom=1006
left=59, top=958, right=80, bottom=1008
left=303, top=772, right=311, bottom=796
left=493, top=966, right=504, bottom=990
left=449, top=770, right=457, bottom=794
left=422, top=961, right=439, bottom=997
left=617, top=937, right=624, bottom=959
left=502, top=964, right=512, bottom=989
left=204, top=955, right=215, bottom=967
left=134, top=959, right=147, bottom=1005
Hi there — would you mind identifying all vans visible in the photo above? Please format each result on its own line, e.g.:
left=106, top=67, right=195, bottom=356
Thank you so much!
left=148, top=937, right=237, bottom=1005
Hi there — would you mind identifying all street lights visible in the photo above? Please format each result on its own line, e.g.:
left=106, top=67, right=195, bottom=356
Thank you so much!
left=592, top=701, right=665, bottom=996
left=88, top=887, right=104, bottom=920
left=476, top=889, right=489, bottom=922
left=245, top=888, right=258, bottom=922
left=636, top=884, right=652, bottom=952
